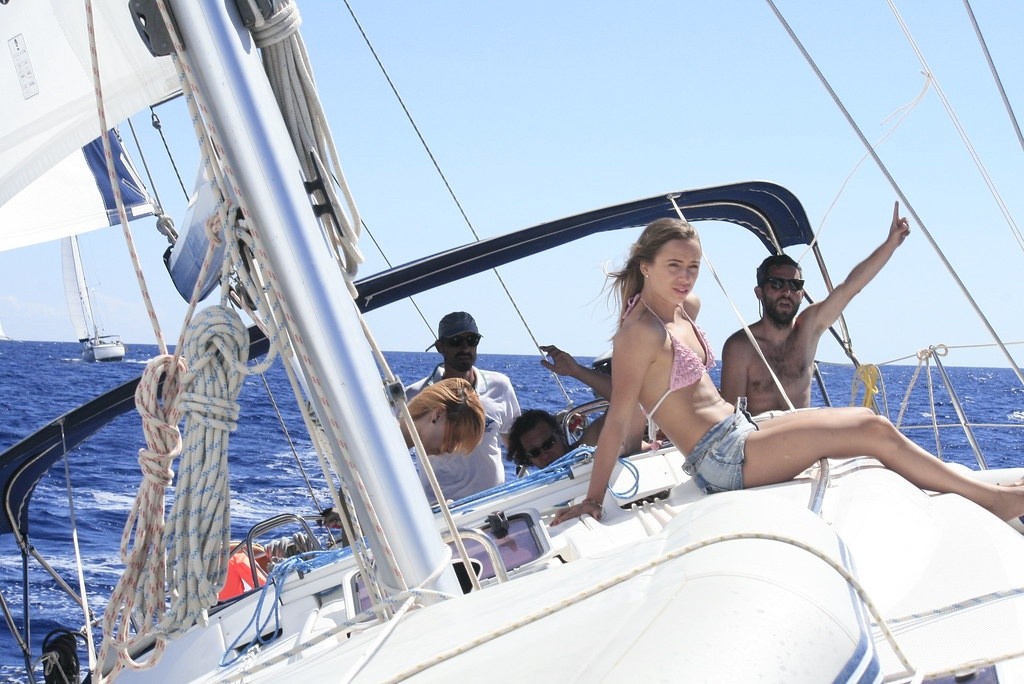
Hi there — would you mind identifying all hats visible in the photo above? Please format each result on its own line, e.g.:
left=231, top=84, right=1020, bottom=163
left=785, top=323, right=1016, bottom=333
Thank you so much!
left=439, top=311, right=484, bottom=338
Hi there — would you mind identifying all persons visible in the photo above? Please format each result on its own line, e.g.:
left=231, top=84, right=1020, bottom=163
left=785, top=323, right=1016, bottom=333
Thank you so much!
left=720, top=201, right=911, bottom=416
left=398, top=377, right=485, bottom=458
left=506, top=345, right=676, bottom=510
left=403, top=311, right=522, bottom=507
left=550, top=218, right=1024, bottom=526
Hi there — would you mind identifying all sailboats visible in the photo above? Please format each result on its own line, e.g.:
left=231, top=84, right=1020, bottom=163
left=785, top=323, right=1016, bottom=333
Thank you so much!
left=0, top=0, right=1024, bottom=684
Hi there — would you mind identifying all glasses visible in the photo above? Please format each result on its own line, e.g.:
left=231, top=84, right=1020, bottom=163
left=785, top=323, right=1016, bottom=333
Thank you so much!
left=525, top=429, right=556, bottom=458
left=444, top=335, right=481, bottom=346
left=760, top=277, right=805, bottom=292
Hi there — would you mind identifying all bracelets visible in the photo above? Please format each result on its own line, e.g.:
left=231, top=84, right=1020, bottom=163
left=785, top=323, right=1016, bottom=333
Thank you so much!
left=581, top=499, right=607, bottom=520
